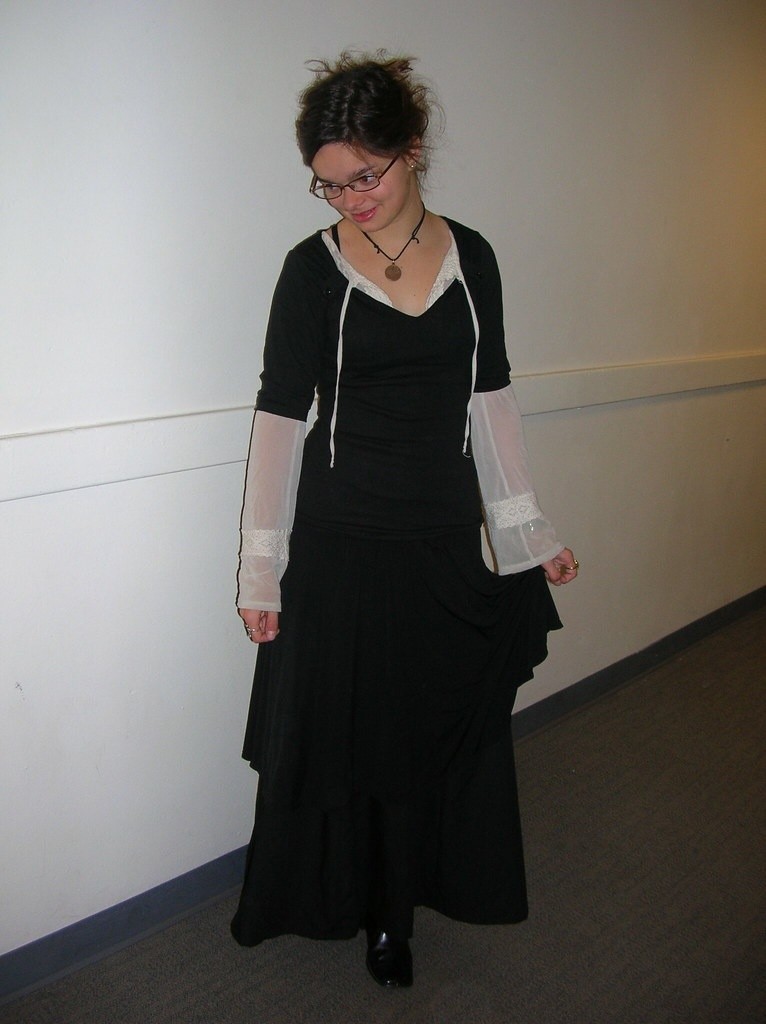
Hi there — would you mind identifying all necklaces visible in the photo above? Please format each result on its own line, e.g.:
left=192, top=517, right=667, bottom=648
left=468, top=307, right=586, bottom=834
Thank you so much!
left=361, top=201, right=426, bottom=281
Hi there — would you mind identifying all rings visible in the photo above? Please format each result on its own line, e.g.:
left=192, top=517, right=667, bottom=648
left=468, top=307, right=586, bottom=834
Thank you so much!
left=566, top=560, right=579, bottom=570
left=244, top=623, right=260, bottom=637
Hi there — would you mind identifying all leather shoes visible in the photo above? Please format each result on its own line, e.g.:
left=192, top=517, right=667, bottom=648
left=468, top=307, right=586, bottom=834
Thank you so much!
left=366, top=921, right=414, bottom=989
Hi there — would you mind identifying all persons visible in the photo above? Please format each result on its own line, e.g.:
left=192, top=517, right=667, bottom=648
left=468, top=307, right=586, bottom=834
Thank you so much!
left=230, top=48, right=580, bottom=986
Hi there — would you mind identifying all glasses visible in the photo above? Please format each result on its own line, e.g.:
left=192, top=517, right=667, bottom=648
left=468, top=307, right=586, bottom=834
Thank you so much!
left=309, top=151, right=403, bottom=200
